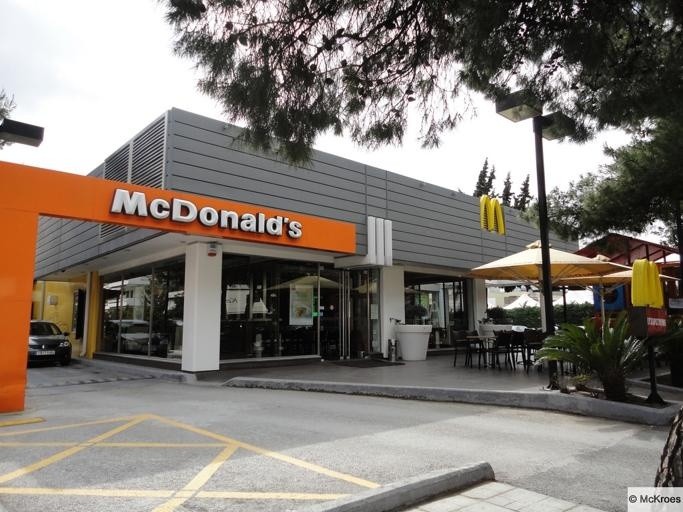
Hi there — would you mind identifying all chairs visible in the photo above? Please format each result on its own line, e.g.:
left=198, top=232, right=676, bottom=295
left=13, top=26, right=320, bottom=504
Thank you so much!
left=452, top=330, right=547, bottom=373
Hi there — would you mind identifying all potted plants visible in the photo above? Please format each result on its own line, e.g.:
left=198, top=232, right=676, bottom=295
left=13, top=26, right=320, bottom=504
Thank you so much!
left=479, top=305, right=513, bottom=336
left=535, top=309, right=682, bottom=400
left=390, top=305, right=432, bottom=361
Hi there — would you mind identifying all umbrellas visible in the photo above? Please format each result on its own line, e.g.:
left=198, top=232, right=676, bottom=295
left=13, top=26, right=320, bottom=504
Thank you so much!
left=264, top=272, right=425, bottom=295
left=470, top=238, right=681, bottom=368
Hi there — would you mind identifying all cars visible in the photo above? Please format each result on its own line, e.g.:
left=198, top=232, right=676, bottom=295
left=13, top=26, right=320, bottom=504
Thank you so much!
left=107, top=319, right=161, bottom=354
left=28, top=320, right=72, bottom=365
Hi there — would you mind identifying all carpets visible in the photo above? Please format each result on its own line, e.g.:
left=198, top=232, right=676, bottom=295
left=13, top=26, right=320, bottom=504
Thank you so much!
left=334, top=358, right=405, bottom=369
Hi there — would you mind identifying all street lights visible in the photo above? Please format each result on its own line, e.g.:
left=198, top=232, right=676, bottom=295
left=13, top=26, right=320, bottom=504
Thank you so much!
left=495, top=89, right=575, bottom=394
left=0, top=113, right=43, bottom=146
